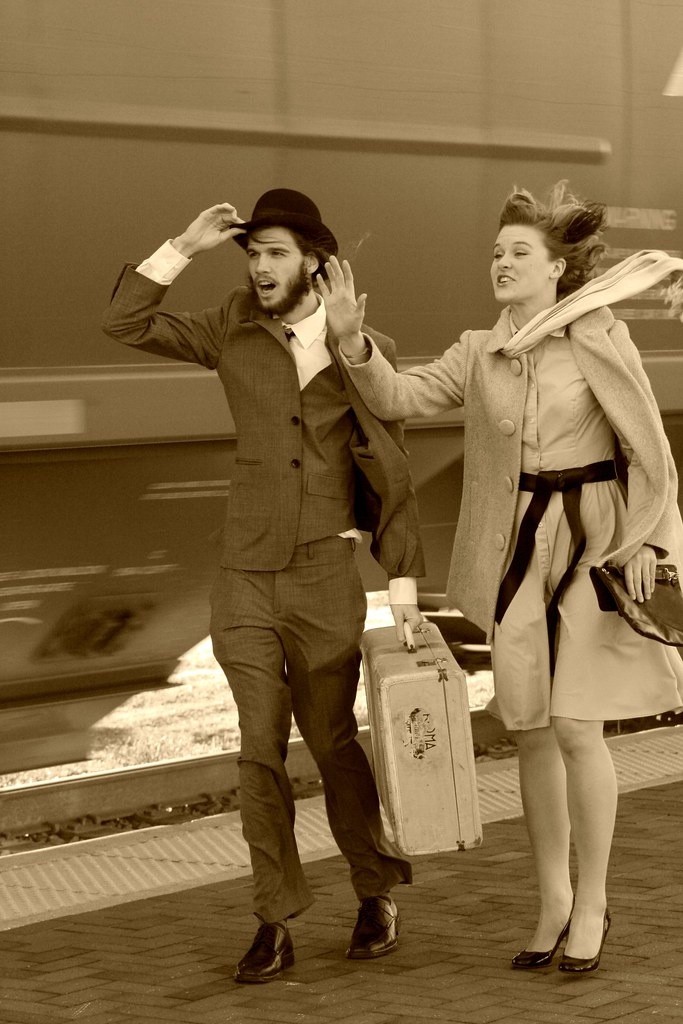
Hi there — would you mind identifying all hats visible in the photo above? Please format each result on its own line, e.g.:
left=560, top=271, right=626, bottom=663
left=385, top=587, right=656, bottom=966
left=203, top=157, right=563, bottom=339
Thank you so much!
left=229, top=188, right=338, bottom=282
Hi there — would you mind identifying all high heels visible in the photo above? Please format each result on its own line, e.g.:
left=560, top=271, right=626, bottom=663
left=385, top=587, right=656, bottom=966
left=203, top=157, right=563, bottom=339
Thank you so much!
left=511, top=894, right=611, bottom=973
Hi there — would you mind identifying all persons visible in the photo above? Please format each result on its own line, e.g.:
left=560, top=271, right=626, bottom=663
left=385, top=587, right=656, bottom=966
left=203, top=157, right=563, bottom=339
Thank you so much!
left=97, top=188, right=429, bottom=987
left=317, top=180, right=683, bottom=974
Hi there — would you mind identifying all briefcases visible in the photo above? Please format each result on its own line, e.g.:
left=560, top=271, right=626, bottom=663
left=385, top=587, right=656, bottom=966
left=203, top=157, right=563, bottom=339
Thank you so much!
left=361, top=621, right=483, bottom=856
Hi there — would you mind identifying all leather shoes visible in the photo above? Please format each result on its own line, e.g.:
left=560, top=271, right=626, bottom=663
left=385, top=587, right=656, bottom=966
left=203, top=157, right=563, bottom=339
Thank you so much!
left=345, top=894, right=401, bottom=959
left=234, top=923, right=295, bottom=983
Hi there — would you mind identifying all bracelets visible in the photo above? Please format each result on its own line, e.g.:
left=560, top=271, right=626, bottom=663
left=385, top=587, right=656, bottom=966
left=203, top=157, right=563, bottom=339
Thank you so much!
left=340, top=347, right=369, bottom=358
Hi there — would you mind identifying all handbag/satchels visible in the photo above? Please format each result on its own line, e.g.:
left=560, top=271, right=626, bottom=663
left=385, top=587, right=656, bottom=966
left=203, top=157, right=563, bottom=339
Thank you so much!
left=589, top=559, right=683, bottom=646
